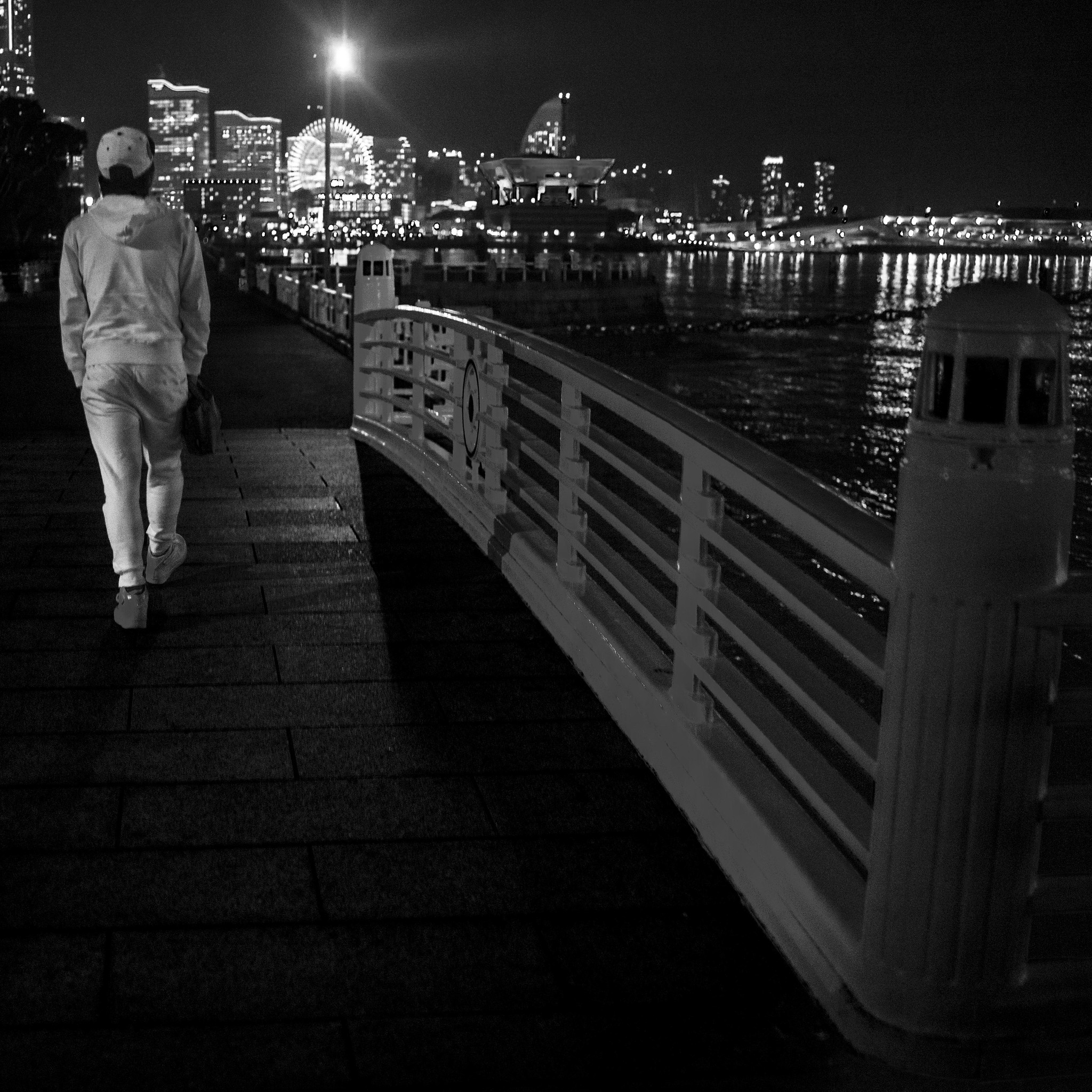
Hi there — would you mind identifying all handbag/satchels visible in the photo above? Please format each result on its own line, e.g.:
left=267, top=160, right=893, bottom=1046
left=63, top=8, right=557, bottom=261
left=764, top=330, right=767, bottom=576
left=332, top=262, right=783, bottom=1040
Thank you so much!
left=183, top=377, right=220, bottom=456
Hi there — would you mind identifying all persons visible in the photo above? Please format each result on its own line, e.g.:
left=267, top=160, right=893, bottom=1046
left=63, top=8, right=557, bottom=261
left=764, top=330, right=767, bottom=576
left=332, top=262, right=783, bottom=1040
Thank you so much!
left=59, top=128, right=210, bottom=629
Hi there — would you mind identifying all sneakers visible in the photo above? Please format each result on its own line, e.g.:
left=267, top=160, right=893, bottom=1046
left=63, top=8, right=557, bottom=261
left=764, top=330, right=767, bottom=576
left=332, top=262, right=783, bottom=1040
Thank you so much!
left=146, top=533, right=187, bottom=584
left=114, top=586, right=148, bottom=629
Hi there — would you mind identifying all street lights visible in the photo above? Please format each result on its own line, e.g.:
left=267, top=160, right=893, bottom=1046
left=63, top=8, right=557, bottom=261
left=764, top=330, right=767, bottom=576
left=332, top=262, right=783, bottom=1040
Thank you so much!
left=321, top=40, right=356, bottom=282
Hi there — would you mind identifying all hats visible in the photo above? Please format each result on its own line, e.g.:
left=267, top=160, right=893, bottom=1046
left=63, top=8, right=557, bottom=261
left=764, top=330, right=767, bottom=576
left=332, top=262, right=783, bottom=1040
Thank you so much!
left=96, top=126, right=156, bottom=182
